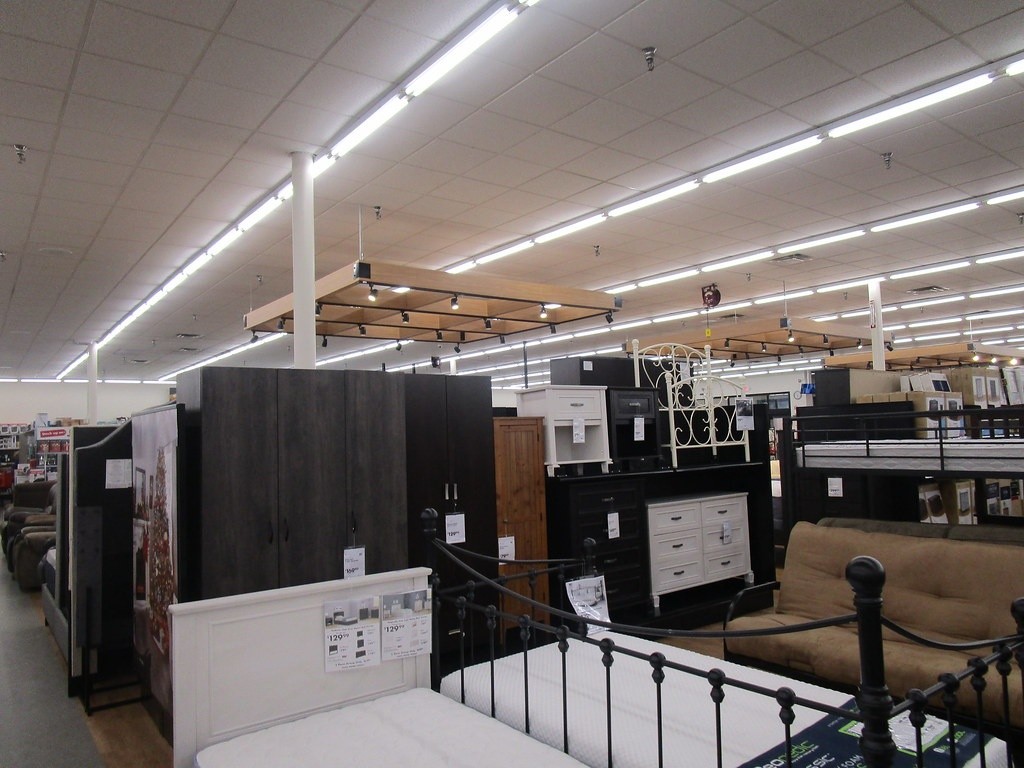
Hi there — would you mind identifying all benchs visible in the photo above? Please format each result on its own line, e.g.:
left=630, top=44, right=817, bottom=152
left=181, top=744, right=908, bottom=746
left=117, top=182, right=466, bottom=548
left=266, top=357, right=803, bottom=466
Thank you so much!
left=721, top=522, right=1024, bottom=730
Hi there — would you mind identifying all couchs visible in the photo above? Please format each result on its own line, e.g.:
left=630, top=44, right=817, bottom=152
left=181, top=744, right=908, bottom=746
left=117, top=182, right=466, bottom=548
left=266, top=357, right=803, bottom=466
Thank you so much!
left=0, top=477, right=59, bottom=593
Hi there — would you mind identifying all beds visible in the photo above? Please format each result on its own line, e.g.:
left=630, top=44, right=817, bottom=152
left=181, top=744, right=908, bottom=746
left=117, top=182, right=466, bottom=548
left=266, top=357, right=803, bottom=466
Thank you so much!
left=170, top=567, right=593, bottom=768
left=418, top=507, right=1024, bottom=768
left=784, top=406, right=1024, bottom=550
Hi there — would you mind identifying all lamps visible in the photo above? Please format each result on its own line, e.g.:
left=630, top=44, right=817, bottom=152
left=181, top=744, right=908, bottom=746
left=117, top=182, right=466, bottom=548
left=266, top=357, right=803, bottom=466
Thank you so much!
left=866, top=353, right=1017, bottom=370
left=705, top=331, right=893, bottom=367
left=250, top=285, right=614, bottom=367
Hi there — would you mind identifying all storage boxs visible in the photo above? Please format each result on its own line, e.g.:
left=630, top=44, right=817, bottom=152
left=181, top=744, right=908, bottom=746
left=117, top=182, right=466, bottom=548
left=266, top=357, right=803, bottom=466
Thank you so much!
left=0, top=425, right=28, bottom=448
left=856, top=365, right=1024, bottom=525
left=14, top=463, right=58, bottom=486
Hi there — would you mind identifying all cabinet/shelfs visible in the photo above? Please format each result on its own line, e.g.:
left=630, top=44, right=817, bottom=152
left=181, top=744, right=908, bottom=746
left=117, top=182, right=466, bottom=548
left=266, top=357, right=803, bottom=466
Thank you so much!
left=34, top=446, right=69, bottom=482
left=177, top=356, right=775, bottom=693
